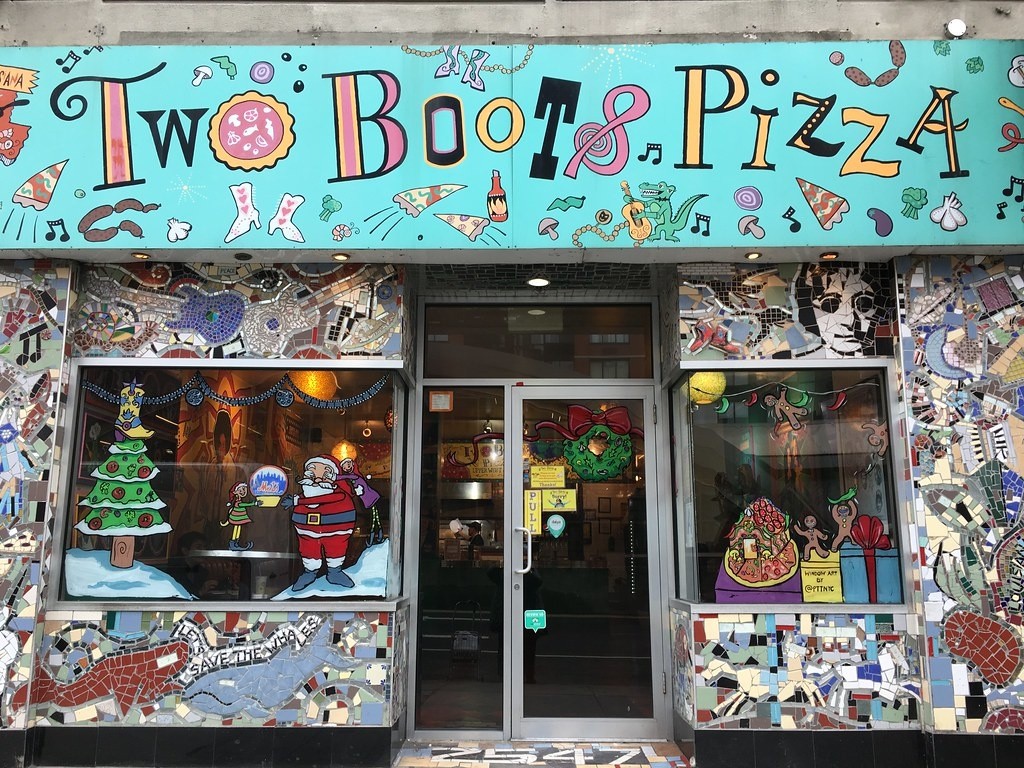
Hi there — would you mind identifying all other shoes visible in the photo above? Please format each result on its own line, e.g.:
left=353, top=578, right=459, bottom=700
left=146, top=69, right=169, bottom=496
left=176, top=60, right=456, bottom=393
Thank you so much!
left=527, top=674, right=537, bottom=685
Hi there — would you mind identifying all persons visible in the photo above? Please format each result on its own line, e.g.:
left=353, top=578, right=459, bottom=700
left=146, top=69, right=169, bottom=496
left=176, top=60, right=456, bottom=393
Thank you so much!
left=422, top=540, right=436, bottom=558
left=489, top=554, right=547, bottom=676
left=164, top=530, right=218, bottom=602
left=459, top=520, right=485, bottom=564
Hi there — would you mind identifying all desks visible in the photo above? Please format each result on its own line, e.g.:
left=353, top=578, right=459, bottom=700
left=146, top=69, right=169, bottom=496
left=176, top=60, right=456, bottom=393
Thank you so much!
left=187, top=548, right=297, bottom=601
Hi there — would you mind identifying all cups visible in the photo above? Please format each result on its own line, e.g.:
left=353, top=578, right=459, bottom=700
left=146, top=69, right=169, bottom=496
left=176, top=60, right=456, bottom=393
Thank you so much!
left=255, top=576, right=267, bottom=594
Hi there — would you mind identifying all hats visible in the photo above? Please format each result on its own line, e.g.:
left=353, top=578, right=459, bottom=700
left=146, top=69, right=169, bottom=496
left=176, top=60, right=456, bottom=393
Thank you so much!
left=466, top=522, right=481, bottom=531
